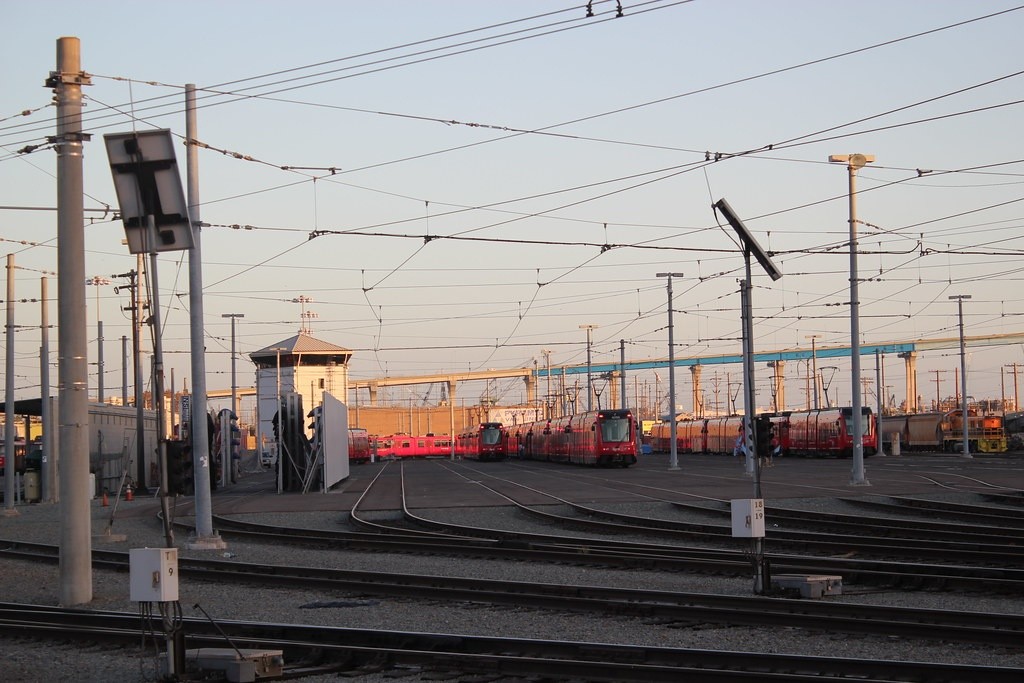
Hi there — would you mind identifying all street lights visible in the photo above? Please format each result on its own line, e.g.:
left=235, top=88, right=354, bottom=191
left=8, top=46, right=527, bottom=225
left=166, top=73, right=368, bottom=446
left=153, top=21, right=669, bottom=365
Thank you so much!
left=806, top=335, right=822, bottom=410
left=578, top=324, right=599, bottom=412
left=949, top=294, right=971, bottom=456
left=657, top=272, right=683, bottom=470
left=269, top=347, right=287, bottom=493
left=829, top=153, right=876, bottom=488
left=222, top=313, right=244, bottom=481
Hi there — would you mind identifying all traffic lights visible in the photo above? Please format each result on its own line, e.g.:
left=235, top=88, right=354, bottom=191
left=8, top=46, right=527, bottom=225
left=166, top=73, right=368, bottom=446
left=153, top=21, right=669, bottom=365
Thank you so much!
left=749, top=417, right=775, bottom=458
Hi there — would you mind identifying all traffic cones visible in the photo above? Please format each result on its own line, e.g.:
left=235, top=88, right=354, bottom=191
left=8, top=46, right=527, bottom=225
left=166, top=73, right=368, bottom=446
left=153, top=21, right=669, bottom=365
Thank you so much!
left=124, top=484, right=135, bottom=501
left=102, top=493, right=109, bottom=506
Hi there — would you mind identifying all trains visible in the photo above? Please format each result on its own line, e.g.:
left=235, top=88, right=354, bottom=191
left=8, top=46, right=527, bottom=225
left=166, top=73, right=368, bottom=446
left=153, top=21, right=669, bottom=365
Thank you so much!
left=348, top=409, right=638, bottom=469
left=0, top=436, right=27, bottom=469
left=879, top=408, right=1024, bottom=453
left=652, top=406, right=878, bottom=459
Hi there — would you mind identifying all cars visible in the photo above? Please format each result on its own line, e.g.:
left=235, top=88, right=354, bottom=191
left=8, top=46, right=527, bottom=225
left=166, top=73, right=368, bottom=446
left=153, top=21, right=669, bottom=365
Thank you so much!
left=263, top=452, right=275, bottom=465
